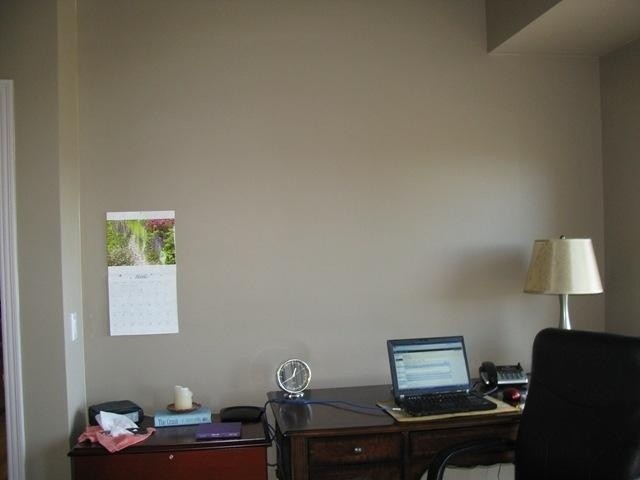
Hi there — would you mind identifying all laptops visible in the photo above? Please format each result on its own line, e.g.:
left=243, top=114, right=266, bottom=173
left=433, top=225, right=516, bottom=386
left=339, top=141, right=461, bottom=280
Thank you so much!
left=387, top=336, right=497, bottom=416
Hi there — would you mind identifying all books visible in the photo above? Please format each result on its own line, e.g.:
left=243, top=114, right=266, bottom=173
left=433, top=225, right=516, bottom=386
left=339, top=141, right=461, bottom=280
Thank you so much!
left=193, top=421, right=244, bottom=441
left=153, top=407, right=213, bottom=428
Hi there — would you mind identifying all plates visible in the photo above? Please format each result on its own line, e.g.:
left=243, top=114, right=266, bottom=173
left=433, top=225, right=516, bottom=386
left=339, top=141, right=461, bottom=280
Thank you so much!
left=167, top=402, right=200, bottom=413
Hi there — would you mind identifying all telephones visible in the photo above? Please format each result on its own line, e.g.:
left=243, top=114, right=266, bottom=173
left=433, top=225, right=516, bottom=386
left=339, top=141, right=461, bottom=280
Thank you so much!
left=479, top=361, right=529, bottom=387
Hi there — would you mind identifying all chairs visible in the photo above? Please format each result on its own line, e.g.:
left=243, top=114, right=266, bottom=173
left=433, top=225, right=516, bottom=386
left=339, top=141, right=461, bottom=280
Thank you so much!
left=422, top=328, right=640, bottom=480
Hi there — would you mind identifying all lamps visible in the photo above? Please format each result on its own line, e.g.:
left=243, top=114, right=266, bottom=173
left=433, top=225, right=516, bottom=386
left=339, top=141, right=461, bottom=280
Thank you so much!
left=521, top=233, right=606, bottom=330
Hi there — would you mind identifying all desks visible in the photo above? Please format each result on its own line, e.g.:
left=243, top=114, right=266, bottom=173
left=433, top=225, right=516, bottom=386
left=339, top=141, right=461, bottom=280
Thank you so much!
left=69, top=406, right=272, bottom=478
left=265, top=370, right=532, bottom=479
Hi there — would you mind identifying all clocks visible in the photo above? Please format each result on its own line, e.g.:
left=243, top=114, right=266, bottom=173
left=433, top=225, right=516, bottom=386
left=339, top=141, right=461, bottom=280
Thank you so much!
left=277, top=356, right=313, bottom=396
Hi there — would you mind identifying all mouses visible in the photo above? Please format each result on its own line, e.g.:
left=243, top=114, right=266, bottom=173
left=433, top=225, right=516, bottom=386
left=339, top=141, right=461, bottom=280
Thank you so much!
left=504, top=388, right=522, bottom=401
